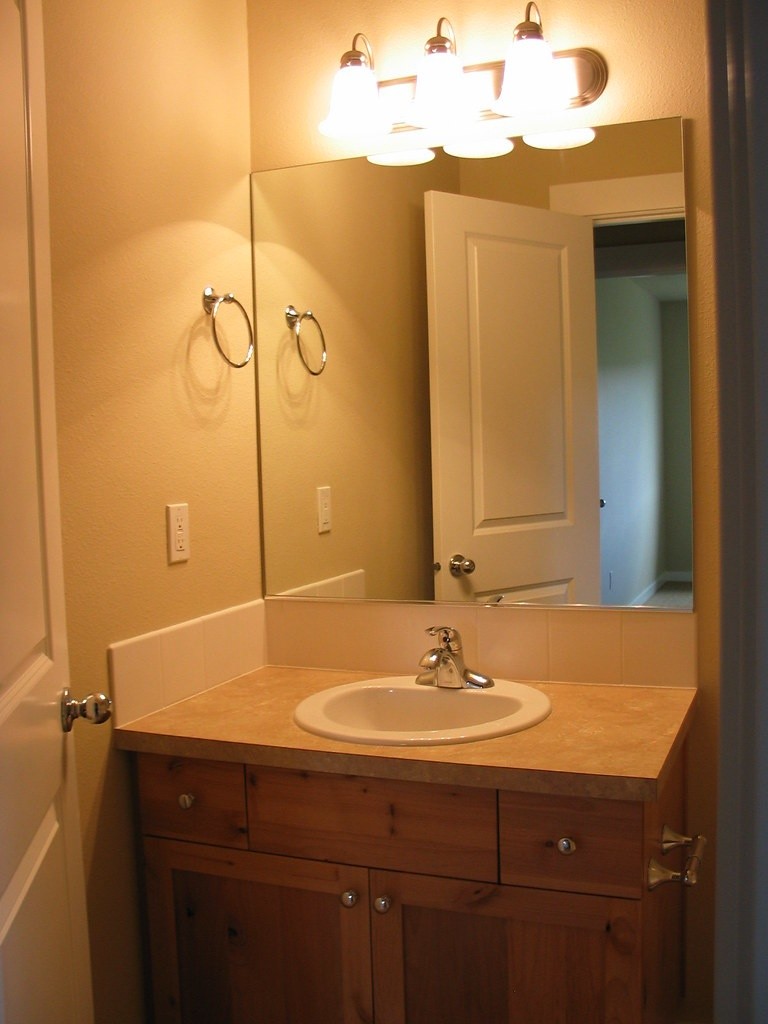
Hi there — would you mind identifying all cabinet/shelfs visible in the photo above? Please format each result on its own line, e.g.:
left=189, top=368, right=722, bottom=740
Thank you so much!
left=139, top=740, right=707, bottom=1024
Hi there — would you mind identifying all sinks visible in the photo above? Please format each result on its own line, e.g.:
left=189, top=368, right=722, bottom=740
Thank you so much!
left=292, top=674, right=553, bottom=747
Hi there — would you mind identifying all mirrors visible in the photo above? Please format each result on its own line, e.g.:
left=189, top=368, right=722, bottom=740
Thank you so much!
left=252, top=116, right=697, bottom=612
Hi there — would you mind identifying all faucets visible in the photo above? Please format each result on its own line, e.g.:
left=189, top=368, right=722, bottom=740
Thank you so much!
left=483, top=595, right=504, bottom=606
left=415, top=624, right=494, bottom=689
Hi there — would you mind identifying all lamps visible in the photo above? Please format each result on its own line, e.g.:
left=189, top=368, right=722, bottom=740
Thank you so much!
left=317, top=1, right=608, bottom=144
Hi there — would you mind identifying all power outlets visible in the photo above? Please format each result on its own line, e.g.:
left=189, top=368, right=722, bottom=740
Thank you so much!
left=166, top=503, right=190, bottom=565
left=317, top=486, right=332, bottom=534
